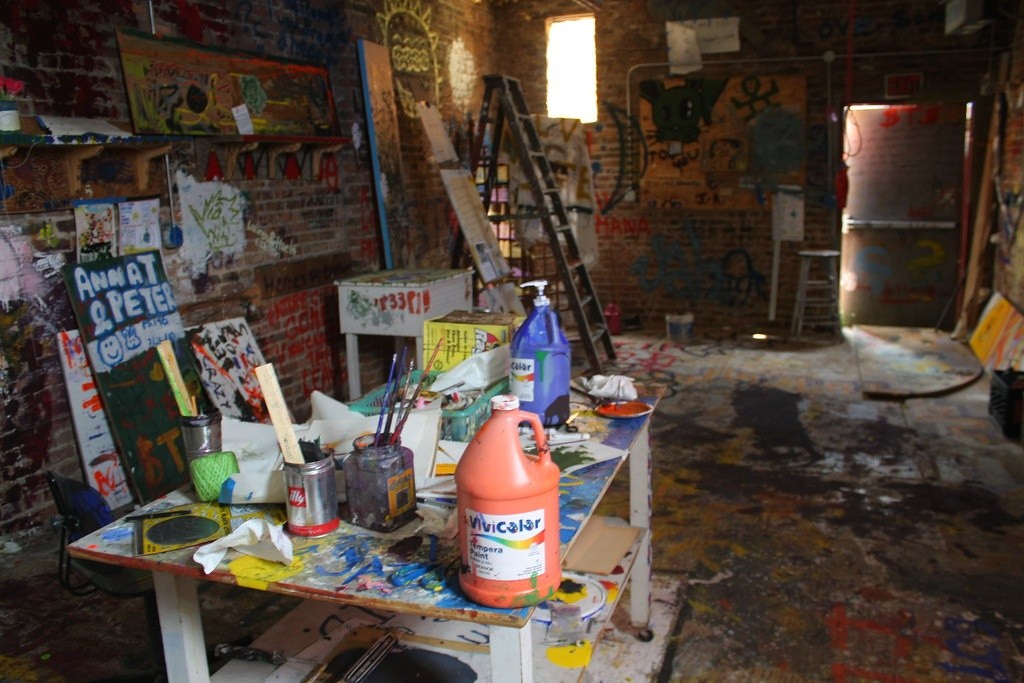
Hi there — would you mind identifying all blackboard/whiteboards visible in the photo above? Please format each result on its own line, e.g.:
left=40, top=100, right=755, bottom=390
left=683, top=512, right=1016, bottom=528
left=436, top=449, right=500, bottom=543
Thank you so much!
left=66, top=250, right=211, bottom=506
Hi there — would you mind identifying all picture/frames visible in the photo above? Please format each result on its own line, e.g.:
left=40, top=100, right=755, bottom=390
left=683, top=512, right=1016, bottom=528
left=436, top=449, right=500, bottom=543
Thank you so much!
left=113, top=25, right=344, bottom=139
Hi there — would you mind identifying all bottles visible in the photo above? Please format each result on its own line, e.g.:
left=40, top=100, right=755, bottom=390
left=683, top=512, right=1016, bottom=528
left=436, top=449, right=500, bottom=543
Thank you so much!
left=486, top=282, right=507, bottom=314
left=603, top=303, right=621, bottom=335
left=476, top=278, right=491, bottom=313
left=343, top=433, right=417, bottom=532
left=454, top=394, right=562, bottom=608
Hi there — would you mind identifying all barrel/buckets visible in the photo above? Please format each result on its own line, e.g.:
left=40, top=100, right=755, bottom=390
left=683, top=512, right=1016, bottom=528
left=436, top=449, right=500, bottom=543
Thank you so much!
left=665, top=311, right=694, bottom=343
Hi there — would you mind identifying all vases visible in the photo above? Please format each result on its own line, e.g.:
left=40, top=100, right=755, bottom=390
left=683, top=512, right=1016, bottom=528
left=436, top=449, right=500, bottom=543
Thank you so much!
left=0, top=100, right=21, bottom=132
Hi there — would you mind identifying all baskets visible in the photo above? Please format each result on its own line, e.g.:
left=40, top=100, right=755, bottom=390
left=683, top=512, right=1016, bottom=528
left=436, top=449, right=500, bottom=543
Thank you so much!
left=348, top=370, right=510, bottom=442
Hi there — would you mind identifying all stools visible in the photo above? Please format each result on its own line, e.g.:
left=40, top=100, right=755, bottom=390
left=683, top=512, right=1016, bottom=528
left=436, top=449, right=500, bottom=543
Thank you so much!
left=790, top=249, right=842, bottom=342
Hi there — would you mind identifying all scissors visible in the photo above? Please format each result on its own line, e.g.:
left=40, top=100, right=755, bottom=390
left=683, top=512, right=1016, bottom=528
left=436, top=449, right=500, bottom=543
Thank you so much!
left=556, top=412, right=579, bottom=432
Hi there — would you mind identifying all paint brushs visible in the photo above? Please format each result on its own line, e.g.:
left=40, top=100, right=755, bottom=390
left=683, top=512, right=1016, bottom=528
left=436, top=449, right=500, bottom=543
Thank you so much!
left=375, top=338, right=446, bottom=445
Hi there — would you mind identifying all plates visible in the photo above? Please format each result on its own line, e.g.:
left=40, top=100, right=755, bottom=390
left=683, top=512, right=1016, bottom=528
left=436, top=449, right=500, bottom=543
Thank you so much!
left=594, top=400, right=654, bottom=419
left=531, top=571, right=607, bottom=623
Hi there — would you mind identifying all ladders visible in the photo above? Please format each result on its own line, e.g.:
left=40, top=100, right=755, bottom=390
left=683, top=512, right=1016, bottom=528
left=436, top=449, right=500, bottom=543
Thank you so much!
left=789, top=249, right=841, bottom=340
left=449, top=75, right=618, bottom=376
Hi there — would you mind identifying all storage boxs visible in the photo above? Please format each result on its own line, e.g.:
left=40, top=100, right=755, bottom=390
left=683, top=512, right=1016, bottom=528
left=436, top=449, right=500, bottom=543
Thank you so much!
left=351, top=370, right=510, bottom=441
left=422, top=309, right=527, bottom=372
left=987, top=370, right=1024, bottom=439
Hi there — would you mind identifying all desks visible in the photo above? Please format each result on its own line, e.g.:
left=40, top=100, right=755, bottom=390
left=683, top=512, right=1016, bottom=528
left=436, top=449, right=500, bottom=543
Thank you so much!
left=70, top=375, right=668, bottom=683
left=333, top=266, right=475, bottom=402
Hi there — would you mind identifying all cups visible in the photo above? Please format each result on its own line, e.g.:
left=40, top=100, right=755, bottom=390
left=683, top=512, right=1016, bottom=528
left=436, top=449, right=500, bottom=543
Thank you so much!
left=177, top=409, right=223, bottom=487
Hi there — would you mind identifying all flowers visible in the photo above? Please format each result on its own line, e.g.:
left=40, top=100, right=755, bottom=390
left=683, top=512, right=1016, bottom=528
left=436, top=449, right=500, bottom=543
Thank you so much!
left=0, top=75, right=24, bottom=100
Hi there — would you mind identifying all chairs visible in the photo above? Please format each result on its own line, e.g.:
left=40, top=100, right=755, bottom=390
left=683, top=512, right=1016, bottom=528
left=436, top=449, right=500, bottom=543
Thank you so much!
left=48, top=470, right=254, bottom=683
